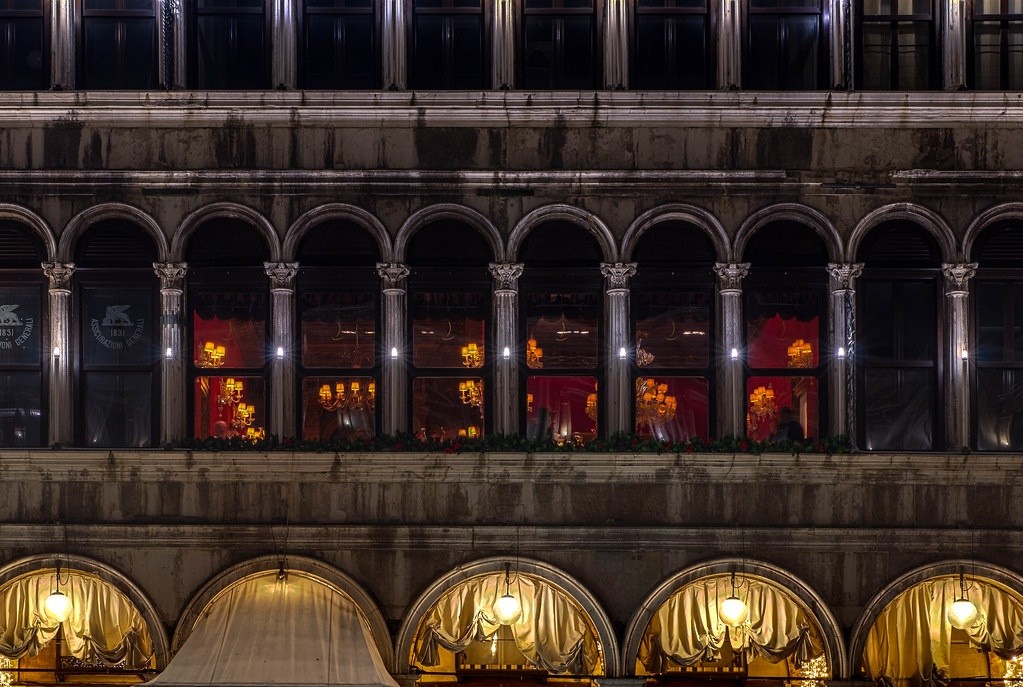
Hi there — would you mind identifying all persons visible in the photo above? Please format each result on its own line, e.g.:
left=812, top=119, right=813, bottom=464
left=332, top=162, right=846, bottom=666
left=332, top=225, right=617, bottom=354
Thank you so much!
left=776, top=407, right=804, bottom=441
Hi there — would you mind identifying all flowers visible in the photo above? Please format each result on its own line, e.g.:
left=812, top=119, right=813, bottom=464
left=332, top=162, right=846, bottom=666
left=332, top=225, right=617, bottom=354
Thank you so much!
left=165, top=431, right=857, bottom=459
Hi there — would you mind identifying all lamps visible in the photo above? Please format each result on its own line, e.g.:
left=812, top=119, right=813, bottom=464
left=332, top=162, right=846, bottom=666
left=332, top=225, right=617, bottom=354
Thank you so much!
left=719, top=567, right=747, bottom=626
left=493, top=563, right=521, bottom=625
left=216, top=378, right=244, bottom=413
left=459, top=380, right=484, bottom=413
left=585, top=383, right=597, bottom=422
left=245, top=427, right=265, bottom=439
left=527, top=339, right=543, bottom=367
left=44, top=559, right=73, bottom=622
left=230, top=403, right=256, bottom=429
left=637, top=378, right=676, bottom=425
left=195, top=342, right=227, bottom=367
left=317, top=380, right=375, bottom=412
left=788, top=339, right=814, bottom=369
left=278, top=560, right=286, bottom=579
left=747, top=387, right=776, bottom=433
left=461, top=343, right=485, bottom=369
left=635, top=338, right=654, bottom=366
